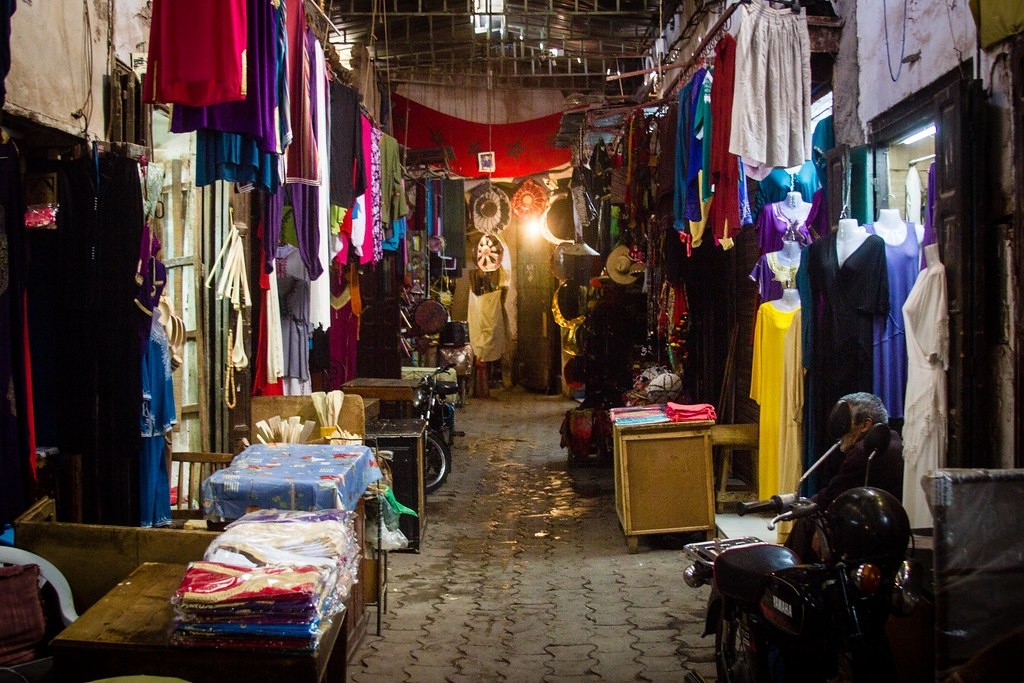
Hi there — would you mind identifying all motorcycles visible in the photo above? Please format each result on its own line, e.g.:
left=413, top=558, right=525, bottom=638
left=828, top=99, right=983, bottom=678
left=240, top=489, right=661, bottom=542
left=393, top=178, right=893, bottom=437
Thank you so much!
left=679, top=400, right=924, bottom=683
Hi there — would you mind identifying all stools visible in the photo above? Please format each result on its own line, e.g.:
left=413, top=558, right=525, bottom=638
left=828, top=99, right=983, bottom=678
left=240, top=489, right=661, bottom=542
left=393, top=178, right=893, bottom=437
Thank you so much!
left=712, top=422, right=761, bottom=513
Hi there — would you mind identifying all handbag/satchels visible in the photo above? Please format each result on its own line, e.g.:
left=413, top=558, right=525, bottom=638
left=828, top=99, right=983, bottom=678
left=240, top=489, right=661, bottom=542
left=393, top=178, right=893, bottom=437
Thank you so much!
left=607, top=168, right=631, bottom=208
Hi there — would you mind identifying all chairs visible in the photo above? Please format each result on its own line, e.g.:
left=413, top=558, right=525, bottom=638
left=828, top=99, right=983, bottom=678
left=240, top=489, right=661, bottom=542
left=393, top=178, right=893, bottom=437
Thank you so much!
left=0, top=545, right=80, bottom=683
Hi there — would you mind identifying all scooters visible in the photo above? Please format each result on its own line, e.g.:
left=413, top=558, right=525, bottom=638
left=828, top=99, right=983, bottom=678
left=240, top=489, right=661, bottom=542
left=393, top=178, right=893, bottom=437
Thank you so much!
left=397, top=362, right=467, bottom=496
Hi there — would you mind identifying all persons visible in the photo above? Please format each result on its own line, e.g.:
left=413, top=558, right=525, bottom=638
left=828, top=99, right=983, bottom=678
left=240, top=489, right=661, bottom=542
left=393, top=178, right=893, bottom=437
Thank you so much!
left=749, top=192, right=948, bottom=529
left=783, top=393, right=904, bottom=564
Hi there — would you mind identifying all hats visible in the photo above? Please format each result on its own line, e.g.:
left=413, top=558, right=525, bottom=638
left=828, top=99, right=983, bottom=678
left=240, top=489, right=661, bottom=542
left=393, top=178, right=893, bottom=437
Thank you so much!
left=606, top=245, right=642, bottom=285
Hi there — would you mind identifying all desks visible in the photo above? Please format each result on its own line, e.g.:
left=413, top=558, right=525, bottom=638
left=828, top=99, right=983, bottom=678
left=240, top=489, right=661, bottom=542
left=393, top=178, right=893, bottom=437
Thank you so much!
left=51, top=560, right=348, bottom=683
left=202, top=442, right=384, bottom=663
left=611, top=404, right=716, bottom=553
left=364, top=418, right=428, bottom=554
left=342, top=377, right=424, bottom=417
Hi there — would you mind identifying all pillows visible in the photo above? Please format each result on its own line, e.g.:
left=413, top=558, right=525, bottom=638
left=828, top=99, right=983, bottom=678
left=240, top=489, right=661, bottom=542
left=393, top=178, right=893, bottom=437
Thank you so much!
left=0, top=563, right=46, bottom=666
